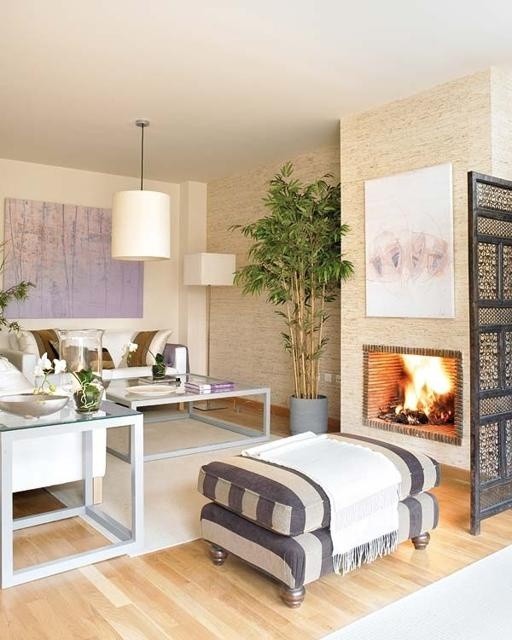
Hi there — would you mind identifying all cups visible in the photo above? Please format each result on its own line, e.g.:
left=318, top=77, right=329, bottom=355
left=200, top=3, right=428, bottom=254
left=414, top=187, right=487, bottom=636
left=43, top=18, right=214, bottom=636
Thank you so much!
left=71, top=385, right=104, bottom=415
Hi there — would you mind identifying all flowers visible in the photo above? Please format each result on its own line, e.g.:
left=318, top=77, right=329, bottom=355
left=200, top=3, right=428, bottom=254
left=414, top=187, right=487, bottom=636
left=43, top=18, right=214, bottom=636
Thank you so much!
left=29, top=351, right=67, bottom=397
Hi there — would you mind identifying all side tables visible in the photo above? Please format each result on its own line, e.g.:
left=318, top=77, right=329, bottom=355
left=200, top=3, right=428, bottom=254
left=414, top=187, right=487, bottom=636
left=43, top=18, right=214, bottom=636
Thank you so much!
left=1, top=391, right=146, bottom=590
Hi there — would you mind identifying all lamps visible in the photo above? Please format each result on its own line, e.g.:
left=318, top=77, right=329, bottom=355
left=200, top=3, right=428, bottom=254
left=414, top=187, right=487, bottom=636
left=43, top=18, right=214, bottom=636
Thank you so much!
left=183, top=253, right=236, bottom=414
left=111, top=120, right=172, bottom=262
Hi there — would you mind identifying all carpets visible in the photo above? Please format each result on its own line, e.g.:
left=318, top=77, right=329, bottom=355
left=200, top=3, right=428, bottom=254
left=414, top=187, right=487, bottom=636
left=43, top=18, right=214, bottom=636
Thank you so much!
left=42, top=411, right=286, bottom=558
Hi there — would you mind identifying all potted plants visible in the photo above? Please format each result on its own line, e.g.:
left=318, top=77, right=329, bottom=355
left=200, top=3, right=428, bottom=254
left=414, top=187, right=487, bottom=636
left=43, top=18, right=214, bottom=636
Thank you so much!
left=227, top=159, right=353, bottom=440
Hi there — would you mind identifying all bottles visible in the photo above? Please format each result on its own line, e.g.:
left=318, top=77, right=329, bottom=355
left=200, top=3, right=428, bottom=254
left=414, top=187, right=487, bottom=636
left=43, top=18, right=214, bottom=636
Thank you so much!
left=175, top=378, right=181, bottom=389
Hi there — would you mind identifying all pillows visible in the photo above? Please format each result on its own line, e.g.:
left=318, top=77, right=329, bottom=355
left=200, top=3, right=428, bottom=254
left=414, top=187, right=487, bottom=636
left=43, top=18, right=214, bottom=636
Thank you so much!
left=16, top=329, right=115, bottom=369
left=119, top=330, right=172, bottom=368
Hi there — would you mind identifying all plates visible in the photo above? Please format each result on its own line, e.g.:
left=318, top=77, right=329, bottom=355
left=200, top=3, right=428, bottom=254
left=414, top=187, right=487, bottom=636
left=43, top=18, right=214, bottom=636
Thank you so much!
left=123, top=385, right=175, bottom=398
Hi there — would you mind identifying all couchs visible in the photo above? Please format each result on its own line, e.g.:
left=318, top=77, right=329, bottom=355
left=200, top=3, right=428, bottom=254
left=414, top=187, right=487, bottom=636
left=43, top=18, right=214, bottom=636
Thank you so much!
left=200, top=429, right=438, bottom=609
left=0, top=326, right=190, bottom=412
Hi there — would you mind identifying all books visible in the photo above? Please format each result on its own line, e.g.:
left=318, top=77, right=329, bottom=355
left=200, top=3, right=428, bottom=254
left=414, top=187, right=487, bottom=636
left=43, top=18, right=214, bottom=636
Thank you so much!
left=183, top=380, right=234, bottom=392
